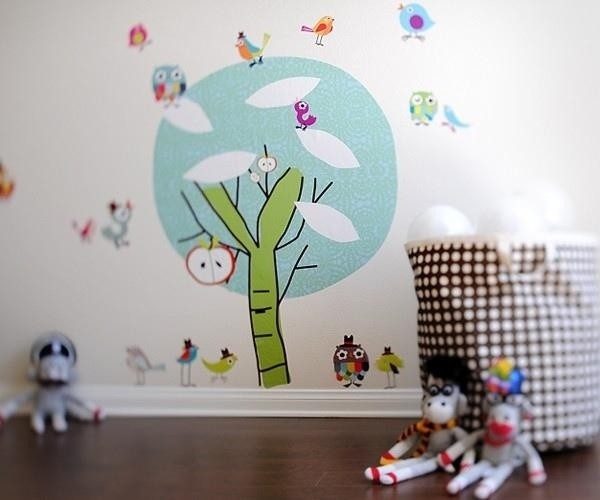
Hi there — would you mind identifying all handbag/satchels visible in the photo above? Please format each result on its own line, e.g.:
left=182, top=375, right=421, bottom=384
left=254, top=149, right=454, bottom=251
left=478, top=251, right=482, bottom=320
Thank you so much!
left=404, top=240, right=599, bottom=454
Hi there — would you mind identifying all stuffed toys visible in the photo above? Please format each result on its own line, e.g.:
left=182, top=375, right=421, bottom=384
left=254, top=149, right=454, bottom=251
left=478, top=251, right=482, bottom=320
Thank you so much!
left=364, top=352, right=475, bottom=487
left=0, top=331, right=107, bottom=435
left=438, top=403, right=547, bottom=499
